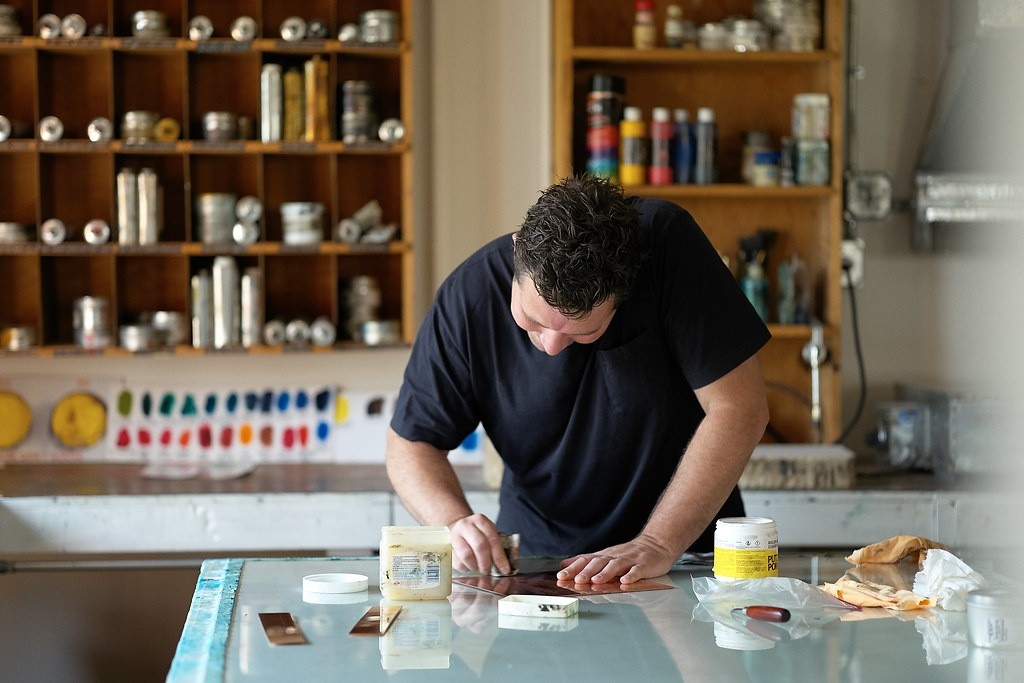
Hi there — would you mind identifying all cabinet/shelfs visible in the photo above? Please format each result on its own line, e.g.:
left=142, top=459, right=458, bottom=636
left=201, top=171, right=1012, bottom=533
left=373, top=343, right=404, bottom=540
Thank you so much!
left=0, top=0, right=435, bottom=359
left=549, top=1, right=852, bottom=447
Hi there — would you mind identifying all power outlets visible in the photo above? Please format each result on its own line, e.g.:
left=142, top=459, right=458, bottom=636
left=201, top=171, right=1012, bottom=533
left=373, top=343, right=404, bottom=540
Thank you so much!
left=839, top=238, right=864, bottom=287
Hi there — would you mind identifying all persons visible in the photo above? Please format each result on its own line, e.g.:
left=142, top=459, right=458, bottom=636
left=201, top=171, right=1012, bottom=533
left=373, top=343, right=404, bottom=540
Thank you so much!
left=386, top=175, right=772, bottom=587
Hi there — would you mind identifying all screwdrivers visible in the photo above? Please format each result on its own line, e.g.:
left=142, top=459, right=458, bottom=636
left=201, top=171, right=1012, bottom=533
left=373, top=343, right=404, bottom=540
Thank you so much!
left=730, top=604, right=791, bottom=623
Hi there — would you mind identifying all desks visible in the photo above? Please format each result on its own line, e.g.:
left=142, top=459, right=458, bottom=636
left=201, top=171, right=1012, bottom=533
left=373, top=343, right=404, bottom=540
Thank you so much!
left=163, top=556, right=1024, bottom=683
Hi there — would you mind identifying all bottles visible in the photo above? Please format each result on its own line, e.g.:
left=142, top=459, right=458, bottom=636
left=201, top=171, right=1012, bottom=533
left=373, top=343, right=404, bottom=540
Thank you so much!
left=137, top=163, right=157, bottom=246
left=304, top=54, right=330, bottom=142
left=241, top=267, right=259, bottom=348
left=212, top=255, right=240, bottom=349
left=350, top=277, right=378, bottom=344
left=586, top=72, right=718, bottom=187
left=284, top=67, right=305, bottom=142
left=737, top=230, right=778, bottom=323
left=74, top=298, right=109, bottom=347
left=282, top=203, right=321, bottom=243
left=201, top=194, right=234, bottom=242
left=663, top=4, right=684, bottom=48
left=740, top=92, right=830, bottom=186
left=632, top=0, right=657, bottom=48
left=260, top=64, right=282, bottom=142
left=117, top=167, right=135, bottom=245
left=777, top=258, right=811, bottom=325
left=191, top=269, right=214, bottom=349
